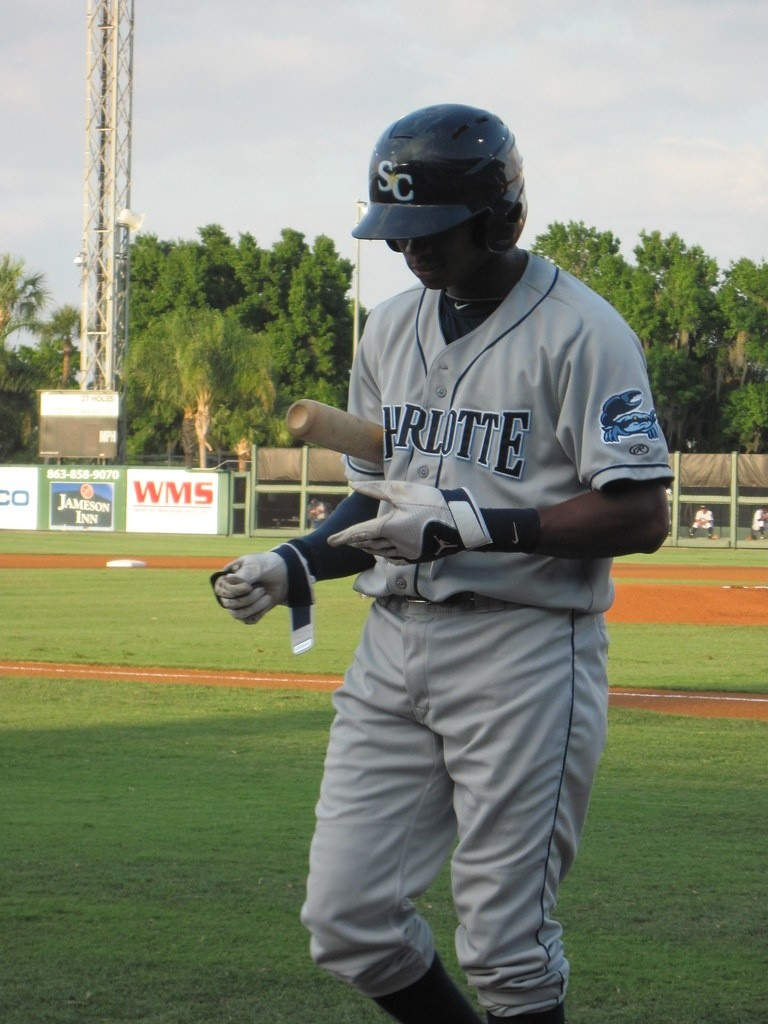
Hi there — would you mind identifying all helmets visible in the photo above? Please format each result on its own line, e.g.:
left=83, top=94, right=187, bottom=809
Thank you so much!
left=352, top=103, right=527, bottom=253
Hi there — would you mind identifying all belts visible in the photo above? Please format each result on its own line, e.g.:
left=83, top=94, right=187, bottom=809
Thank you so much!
left=394, top=592, right=474, bottom=605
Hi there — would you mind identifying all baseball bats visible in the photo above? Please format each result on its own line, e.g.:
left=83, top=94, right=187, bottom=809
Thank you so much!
left=283, top=397, right=385, bottom=464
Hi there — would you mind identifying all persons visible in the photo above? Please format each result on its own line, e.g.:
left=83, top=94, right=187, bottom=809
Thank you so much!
left=689, top=505, right=713, bottom=539
left=752, top=507, right=768, bottom=540
left=210, top=105, right=675, bottom=1024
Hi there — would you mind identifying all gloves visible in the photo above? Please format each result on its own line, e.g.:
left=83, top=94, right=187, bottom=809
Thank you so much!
left=209, top=542, right=317, bottom=654
left=326, top=479, right=493, bottom=566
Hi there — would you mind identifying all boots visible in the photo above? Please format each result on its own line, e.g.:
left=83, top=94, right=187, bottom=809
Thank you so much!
left=488, top=1001, right=566, bottom=1024
left=371, top=950, right=484, bottom=1024
